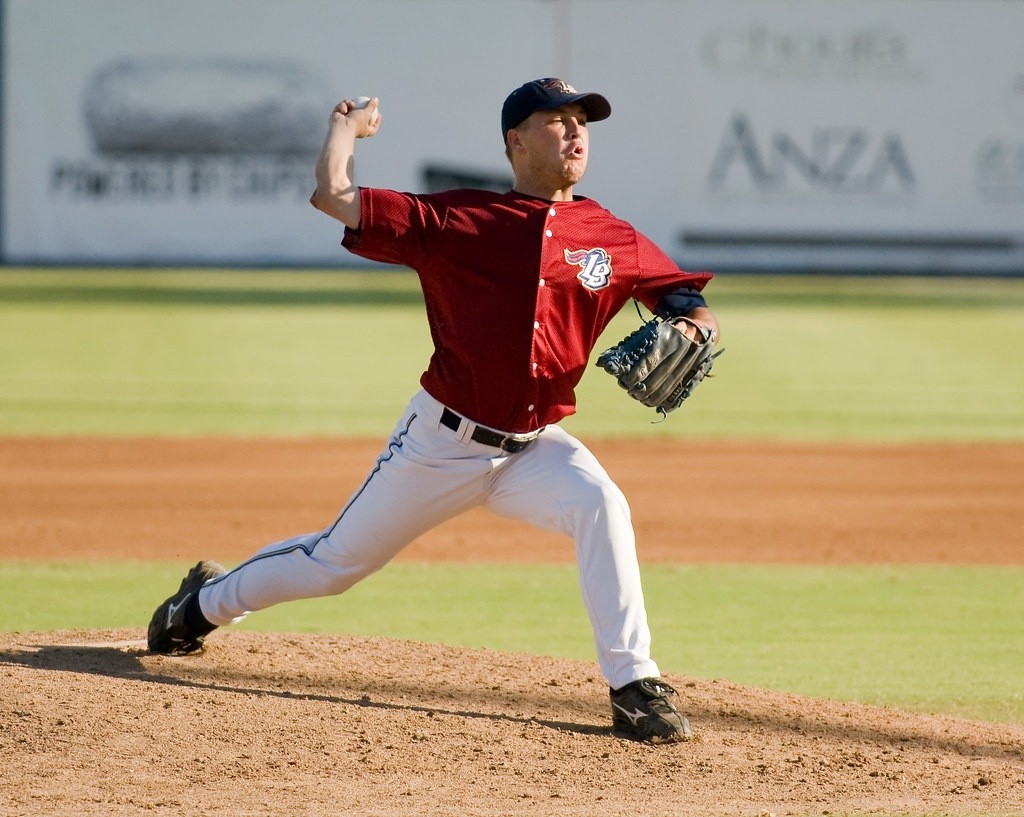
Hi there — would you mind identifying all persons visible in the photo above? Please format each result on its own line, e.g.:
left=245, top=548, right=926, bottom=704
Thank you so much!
left=148, top=77, right=725, bottom=740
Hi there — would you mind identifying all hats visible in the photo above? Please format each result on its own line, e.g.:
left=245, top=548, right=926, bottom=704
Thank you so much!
left=500, top=76, right=612, bottom=144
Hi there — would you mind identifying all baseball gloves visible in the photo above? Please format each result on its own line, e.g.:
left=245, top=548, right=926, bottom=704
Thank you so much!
left=594, top=312, right=725, bottom=415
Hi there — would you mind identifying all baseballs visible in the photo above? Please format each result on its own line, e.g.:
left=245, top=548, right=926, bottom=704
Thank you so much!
left=353, top=93, right=377, bottom=122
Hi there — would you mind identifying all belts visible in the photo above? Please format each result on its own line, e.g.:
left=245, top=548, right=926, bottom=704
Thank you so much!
left=441, top=408, right=545, bottom=453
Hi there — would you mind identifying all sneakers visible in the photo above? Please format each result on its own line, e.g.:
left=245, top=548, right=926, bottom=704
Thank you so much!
left=609, top=676, right=692, bottom=745
left=147, top=560, right=226, bottom=656
